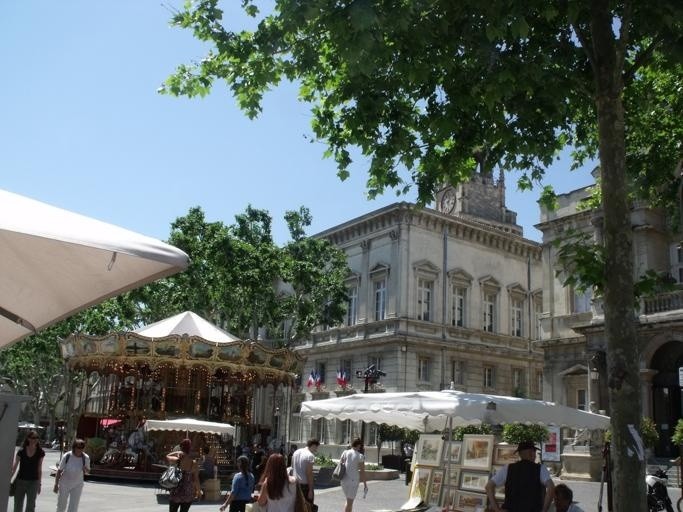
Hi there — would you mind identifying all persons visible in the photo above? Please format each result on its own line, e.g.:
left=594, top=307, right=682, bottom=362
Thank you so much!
left=404, top=444, right=413, bottom=461
left=291, top=438, right=320, bottom=503
left=546, top=483, right=584, bottom=511
left=485, top=441, right=555, bottom=512
left=339, top=438, right=368, bottom=512
left=107, top=425, right=296, bottom=512
left=53, top=439, right=90, bottom=512
left=11, top=430, right=45, bottom=512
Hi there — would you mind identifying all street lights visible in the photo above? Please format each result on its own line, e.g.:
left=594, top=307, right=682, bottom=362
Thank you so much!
left=356, top=365, right=388, bottom=454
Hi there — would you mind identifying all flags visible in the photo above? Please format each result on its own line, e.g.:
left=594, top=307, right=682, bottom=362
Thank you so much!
left=307, top=369, right=321, bottom=391
left=337, top=367, right=349, bottom=390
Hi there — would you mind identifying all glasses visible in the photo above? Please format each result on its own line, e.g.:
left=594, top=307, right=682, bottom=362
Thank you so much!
left=31, top=437, right=39, bottom=439
left=77, top=447, right=84, bottom=449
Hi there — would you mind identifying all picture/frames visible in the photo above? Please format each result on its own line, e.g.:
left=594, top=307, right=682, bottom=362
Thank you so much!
left=409, top=433, right=522, bottom=512
left=540, top=424, right=561, bottom=463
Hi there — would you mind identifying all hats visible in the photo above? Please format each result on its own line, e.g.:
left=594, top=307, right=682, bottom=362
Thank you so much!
left=513, top=442, right=542, bottom=454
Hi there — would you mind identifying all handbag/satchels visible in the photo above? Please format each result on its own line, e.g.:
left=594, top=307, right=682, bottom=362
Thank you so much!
left=159, top=465, right=183, bottom=488
left=9, top=482, right=15, bottom=495
left=296, top=491, right=318, bottom=512
left=156, top=494, right=170, bottom=504
left=331, top=463, right=345, bottom=480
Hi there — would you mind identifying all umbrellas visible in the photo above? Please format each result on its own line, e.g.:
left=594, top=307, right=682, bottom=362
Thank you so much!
left=1, top=187, right=196, bottom=355
left=143, top=417, right=236, bottom=437
left=298, top=381, right=612, bottom=512
